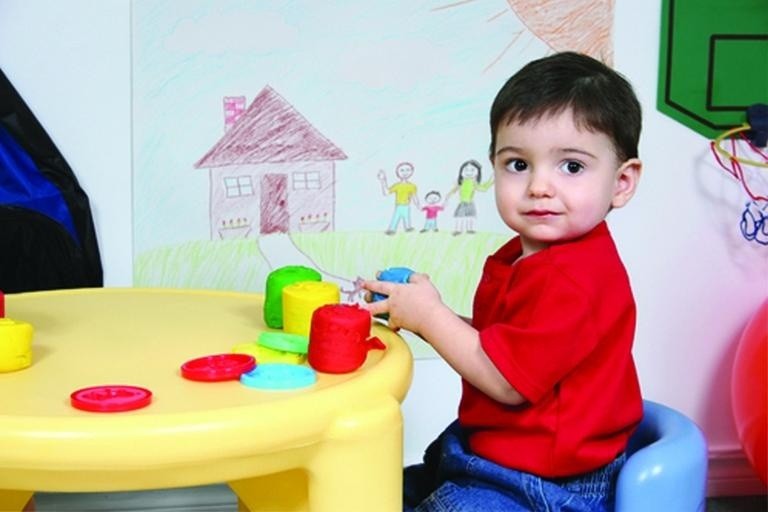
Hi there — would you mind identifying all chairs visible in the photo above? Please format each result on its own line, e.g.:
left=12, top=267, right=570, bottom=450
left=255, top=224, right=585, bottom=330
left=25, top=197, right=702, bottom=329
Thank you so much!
left=613, top=400, right=709, bottom=512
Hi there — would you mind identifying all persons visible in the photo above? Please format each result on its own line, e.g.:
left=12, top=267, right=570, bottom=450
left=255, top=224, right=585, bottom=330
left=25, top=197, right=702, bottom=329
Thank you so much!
left=363, top=50, right=643, bottom=511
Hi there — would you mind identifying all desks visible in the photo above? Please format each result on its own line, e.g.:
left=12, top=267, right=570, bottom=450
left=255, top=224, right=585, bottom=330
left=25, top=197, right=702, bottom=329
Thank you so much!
left=0, top=287, right=416, bottom=512
